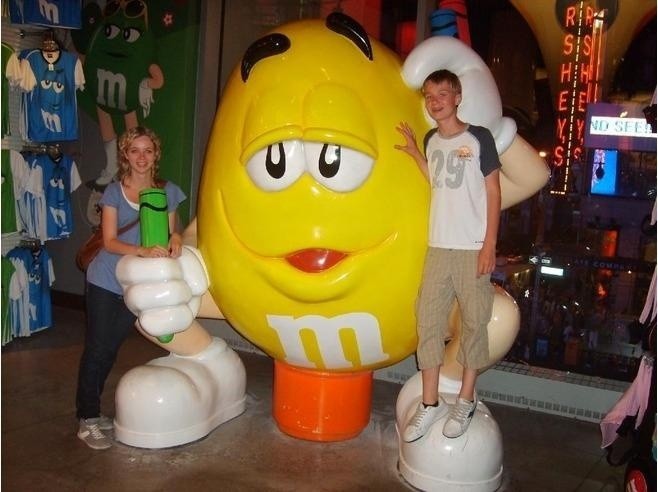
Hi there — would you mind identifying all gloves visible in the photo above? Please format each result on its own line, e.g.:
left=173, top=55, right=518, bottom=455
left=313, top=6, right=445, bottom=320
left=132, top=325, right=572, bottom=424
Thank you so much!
left=139, top=79, right=154, bottom=118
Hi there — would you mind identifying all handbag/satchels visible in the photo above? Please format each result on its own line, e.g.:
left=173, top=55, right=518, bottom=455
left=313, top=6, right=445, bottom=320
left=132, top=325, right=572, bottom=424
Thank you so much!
left=75, top=224, right=105, bottom=270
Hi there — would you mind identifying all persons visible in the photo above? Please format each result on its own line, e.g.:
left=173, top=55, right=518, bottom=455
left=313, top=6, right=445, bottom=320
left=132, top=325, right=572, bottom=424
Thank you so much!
left=73, top=126, right=186, bottom=451
left=393, top=68, right=504, bottom=444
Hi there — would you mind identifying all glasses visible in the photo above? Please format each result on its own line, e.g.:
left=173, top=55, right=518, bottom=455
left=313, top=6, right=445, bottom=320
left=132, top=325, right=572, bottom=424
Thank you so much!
left=102, top=0, right=151, bottom=30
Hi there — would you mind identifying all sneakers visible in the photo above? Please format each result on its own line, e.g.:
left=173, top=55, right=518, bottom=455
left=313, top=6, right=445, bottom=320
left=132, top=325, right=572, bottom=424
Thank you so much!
left=442, top=394, right=477, bottom=439
left=97, top=167, right=118, bottom=185
left=403, top=395, right=449, bottom=444
left=77, top=413, right=112, bottom=451
